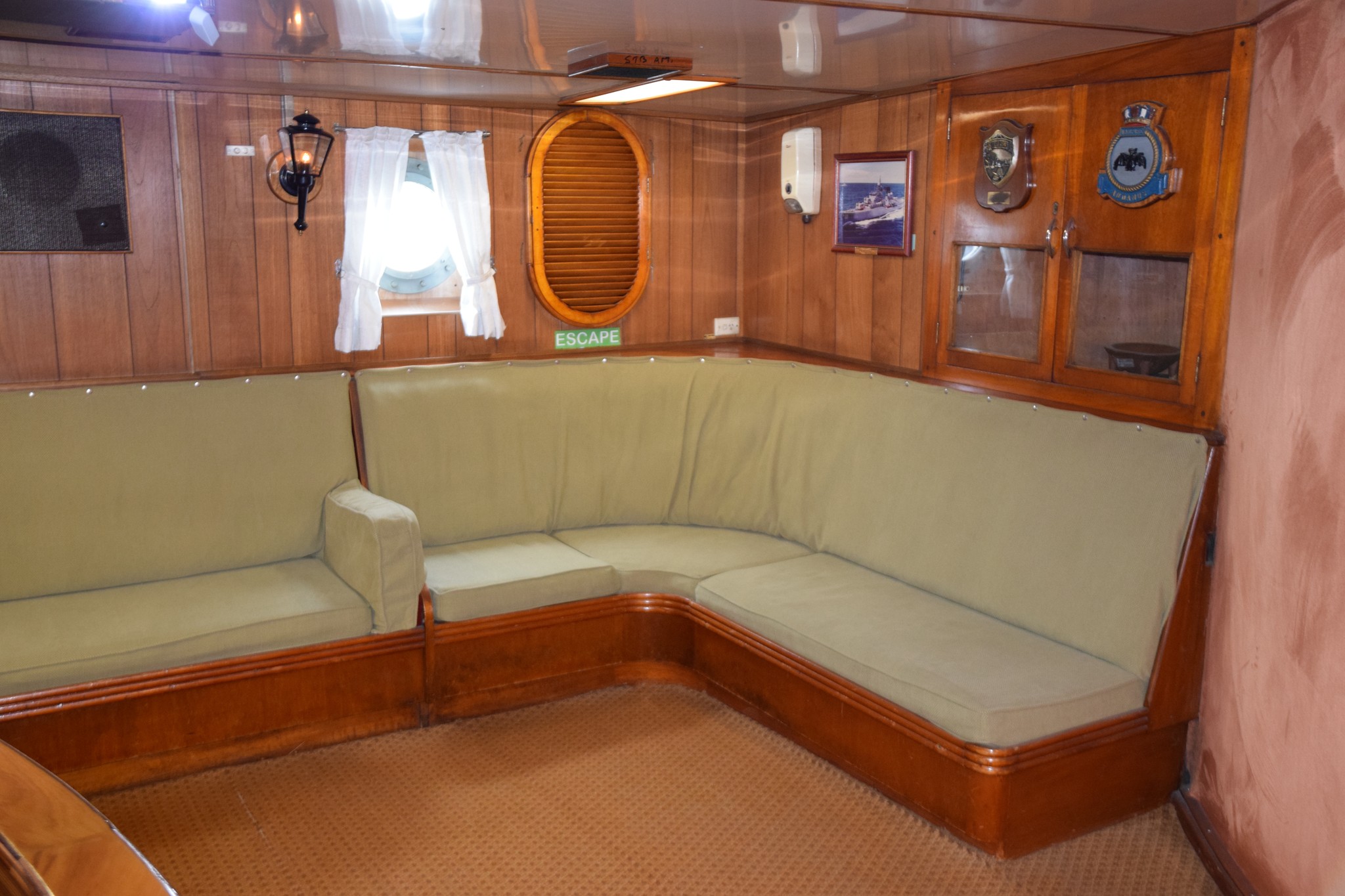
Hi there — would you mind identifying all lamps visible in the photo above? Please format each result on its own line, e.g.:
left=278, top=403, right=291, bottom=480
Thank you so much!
left=266, top=106, right=334, bottom=235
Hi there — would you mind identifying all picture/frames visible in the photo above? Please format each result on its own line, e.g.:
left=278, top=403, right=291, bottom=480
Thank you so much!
left=830, top=150, right=917, bottom=254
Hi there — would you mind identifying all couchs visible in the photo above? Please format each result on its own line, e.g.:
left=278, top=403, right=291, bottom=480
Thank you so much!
left=352, top=332, right=1223, bottom=858
left=0, top=363, right=425, bottom=800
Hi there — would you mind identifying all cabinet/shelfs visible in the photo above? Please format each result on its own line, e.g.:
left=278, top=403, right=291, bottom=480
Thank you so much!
left=919, top=29, right=1256, bottom=429
